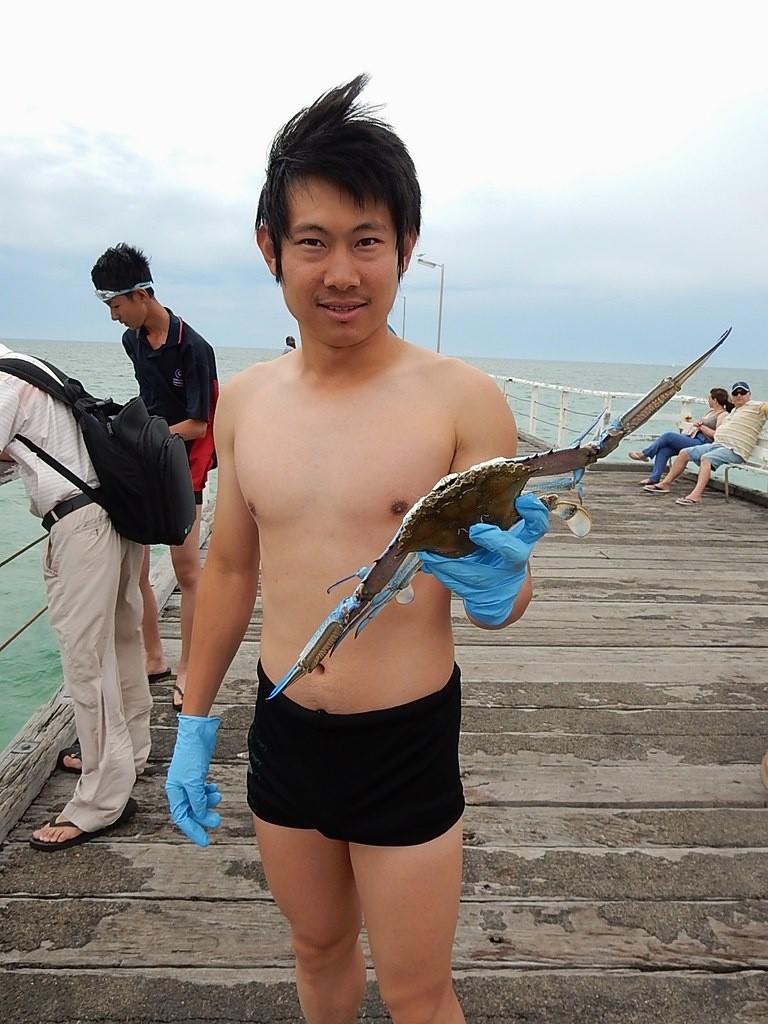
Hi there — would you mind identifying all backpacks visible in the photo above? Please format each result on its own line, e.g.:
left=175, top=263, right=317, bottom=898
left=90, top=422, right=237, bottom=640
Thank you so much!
left=0, top=350, right=196, bottom=547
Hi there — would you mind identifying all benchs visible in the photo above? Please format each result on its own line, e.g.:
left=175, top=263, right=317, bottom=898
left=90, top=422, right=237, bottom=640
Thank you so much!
left=668, top=427, right=768, bottom=504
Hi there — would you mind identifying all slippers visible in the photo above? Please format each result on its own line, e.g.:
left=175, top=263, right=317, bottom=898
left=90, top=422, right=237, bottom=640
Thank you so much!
left=28, top=797, right=139, bottom=853
left=628, top=450, right=650, bottom=462
left=642, top=484, right=671, bottom=493
left=58, top=745, right=161, bottom=776
left=638, top=478, right=660, bottom=485
left=675, top=495, right=704, bottom=506
left=171, top=684, right=187, bottom=713
left=147, top=667, right=172, bottom=683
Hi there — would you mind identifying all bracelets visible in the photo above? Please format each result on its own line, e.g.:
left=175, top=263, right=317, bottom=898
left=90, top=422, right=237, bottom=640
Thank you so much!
left=698, top=422, right=704, bottom=427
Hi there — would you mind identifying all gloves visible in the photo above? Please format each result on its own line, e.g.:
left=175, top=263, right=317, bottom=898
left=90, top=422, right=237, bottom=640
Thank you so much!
left=163, top=712, right=222, bottom=849
left=417, top=493, right=550, bottom=627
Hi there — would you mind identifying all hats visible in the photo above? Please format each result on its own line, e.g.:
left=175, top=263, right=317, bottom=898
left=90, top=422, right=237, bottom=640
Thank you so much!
left=731, top=382, right=750, bottom=393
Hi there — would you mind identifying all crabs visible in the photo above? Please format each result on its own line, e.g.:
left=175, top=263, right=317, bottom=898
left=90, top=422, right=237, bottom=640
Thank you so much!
left=264, top=328, right=734, bottom=702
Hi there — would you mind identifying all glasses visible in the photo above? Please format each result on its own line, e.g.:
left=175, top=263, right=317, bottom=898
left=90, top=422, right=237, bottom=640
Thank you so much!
left=732, top=389, right=749, bottom=396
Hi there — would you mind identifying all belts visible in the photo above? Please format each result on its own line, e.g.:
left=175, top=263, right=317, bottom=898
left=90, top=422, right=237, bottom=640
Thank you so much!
left=40, top=486, right=101, bottom=530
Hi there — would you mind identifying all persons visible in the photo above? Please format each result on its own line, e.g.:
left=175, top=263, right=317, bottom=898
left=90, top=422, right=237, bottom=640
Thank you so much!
left=163, top=73, right=547, bottom=1024
left=0, top=342, right=154, bottom=853
left=90, top=242, right=220, bottom=709
left=282, top=336, right=297, bottom=356
left=629, top=387, right=735, bottom=485
left=642, top=381, right=768, bottom=505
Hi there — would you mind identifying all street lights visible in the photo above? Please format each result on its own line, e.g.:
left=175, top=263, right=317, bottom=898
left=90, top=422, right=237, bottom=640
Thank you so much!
left=417, top=257, right=445, bottom=353
